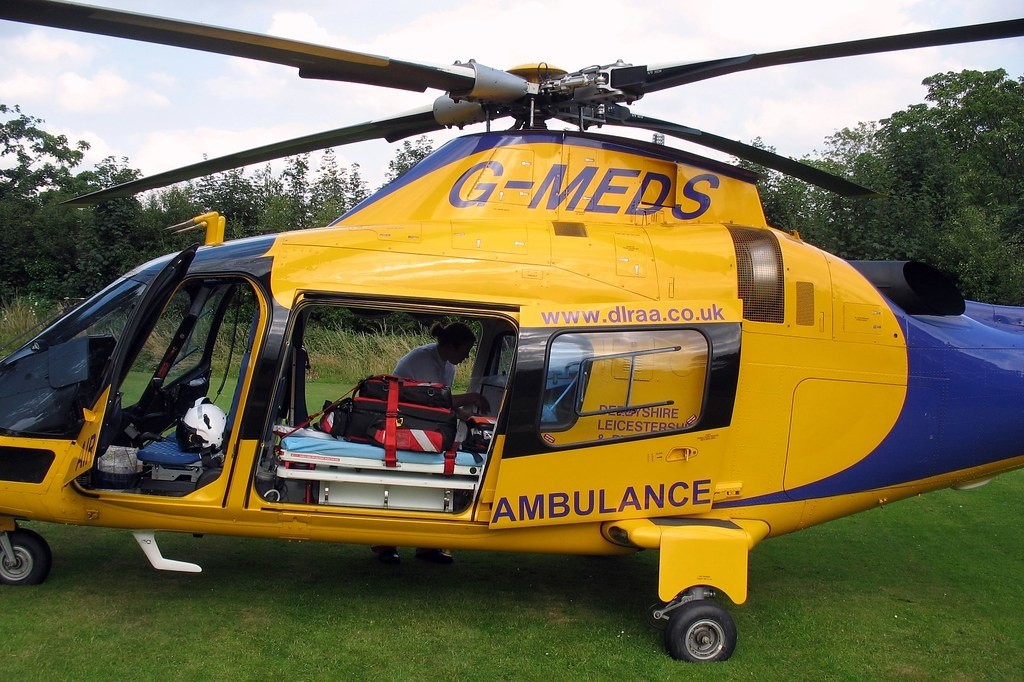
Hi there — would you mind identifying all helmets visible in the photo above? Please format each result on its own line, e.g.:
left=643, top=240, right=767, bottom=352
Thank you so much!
left=176, top=396, right=227, bottom=453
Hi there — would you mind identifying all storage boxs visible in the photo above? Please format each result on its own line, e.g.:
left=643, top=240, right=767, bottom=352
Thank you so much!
left=313, top=468, right=455, bottom=512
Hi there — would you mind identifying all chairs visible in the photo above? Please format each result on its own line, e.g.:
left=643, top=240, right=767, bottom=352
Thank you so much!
left=137, top=306, right=291, bottom=491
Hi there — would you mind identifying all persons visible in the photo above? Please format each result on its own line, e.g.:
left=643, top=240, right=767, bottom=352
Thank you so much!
left=371, top=323, right=491, bottom=570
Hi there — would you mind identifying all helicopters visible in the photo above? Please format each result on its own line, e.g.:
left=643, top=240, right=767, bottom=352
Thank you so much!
left=0, top=0, right=1024, bottom=666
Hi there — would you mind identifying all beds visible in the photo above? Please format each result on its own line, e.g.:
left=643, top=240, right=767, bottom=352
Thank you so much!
left=274, top=432, right=488, bottom=491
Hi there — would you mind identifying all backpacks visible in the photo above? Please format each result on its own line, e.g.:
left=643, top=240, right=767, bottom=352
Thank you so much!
left=312, top=373, right=458, bottom=453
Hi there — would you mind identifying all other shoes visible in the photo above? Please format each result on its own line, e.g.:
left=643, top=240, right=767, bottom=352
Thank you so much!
left=377, top=549, right=401, bottom=566
left=415, top=548, right=454, bottom=565
